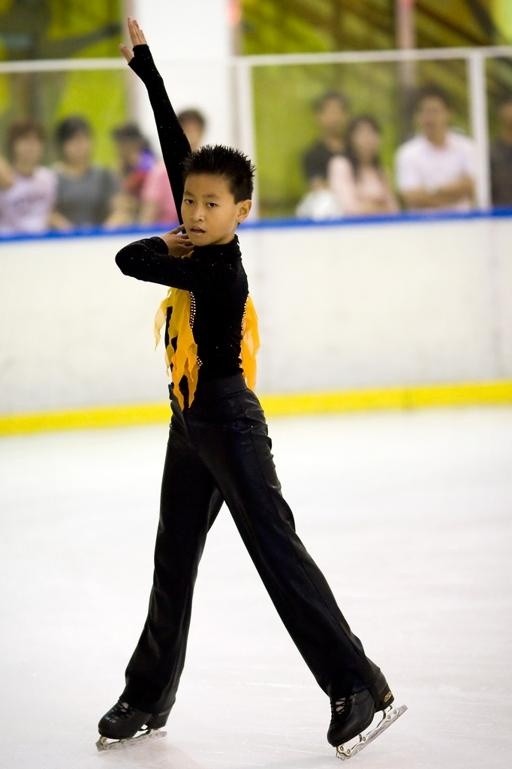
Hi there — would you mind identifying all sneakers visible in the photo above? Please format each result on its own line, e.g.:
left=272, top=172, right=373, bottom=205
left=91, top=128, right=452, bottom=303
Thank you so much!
left=327, top=671, right=394, bottom=746
left=99, top=695, right=170, bottom=738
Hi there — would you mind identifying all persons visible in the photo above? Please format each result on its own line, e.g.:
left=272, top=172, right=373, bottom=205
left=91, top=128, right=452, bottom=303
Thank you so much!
left=298, top=82, right=512, bottom=214
left=0, top=108, right=207, bottom=236
left=94, top=12, right=394, bottom=748
